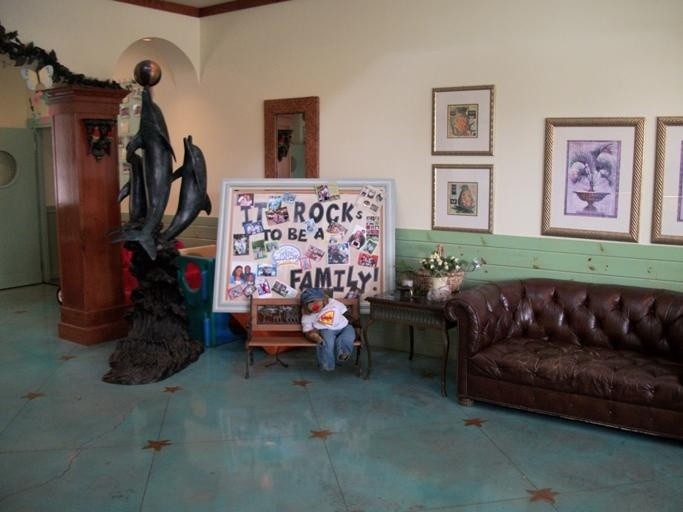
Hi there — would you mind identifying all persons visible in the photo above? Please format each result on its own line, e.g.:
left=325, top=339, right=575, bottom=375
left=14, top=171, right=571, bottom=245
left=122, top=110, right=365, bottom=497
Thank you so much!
left=327, top=243, right=348, bottom=265
left=230, top=265, right=255, bottom=284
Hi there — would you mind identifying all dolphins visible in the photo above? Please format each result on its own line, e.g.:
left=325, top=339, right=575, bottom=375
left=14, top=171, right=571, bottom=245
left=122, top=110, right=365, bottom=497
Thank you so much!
left=456, top=184, right=474, bottom=210
left=112, top=83, right=214, bottom=264
left=568, top=140, right=616, bottom=191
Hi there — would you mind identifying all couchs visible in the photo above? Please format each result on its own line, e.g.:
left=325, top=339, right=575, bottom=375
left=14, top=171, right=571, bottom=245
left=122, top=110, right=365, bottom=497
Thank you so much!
left=444, top=278, right=683, bottom=441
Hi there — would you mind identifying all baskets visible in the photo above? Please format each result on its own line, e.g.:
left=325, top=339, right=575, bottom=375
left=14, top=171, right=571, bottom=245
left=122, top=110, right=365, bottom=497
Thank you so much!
left=413, top=244, right=463, bottom=293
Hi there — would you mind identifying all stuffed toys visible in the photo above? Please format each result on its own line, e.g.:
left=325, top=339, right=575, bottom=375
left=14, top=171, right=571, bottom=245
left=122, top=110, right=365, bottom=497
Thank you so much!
left=298, top=288, right=356, bottom=373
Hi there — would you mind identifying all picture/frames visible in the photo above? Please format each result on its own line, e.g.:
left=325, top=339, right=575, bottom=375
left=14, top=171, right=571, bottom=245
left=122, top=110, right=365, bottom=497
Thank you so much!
left=211, top=176, right=395, bottom=314
left=541, top=118, right=645, bottom=242
left=265, top=97, right=319, bottom=178
left=432, top=85, right=495, bottom=156
left=651, top=117, right=683, bottom=245
left=432, top=164, right=494, bottom=234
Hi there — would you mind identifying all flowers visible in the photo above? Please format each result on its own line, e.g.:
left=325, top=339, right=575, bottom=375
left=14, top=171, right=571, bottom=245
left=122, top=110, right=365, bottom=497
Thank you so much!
left=421, top=252, right=480, bottom=278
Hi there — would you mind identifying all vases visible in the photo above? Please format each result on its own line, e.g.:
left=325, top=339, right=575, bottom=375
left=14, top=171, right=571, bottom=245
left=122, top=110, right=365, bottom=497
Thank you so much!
left=427, top=278, right=454, bottom=302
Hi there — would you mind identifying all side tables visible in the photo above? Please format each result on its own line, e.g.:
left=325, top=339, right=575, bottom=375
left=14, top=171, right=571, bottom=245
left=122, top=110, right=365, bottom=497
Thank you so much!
left=364, top=288, right=457, bottom=398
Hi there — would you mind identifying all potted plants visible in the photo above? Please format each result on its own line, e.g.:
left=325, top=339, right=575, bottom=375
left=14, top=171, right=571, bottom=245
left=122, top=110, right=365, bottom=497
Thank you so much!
left=397, top=259, right=416, bottom=286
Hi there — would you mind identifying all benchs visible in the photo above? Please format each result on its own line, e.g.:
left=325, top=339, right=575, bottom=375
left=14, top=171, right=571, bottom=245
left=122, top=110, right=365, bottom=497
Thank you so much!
left=245, top=294, right=362, bottom=379
